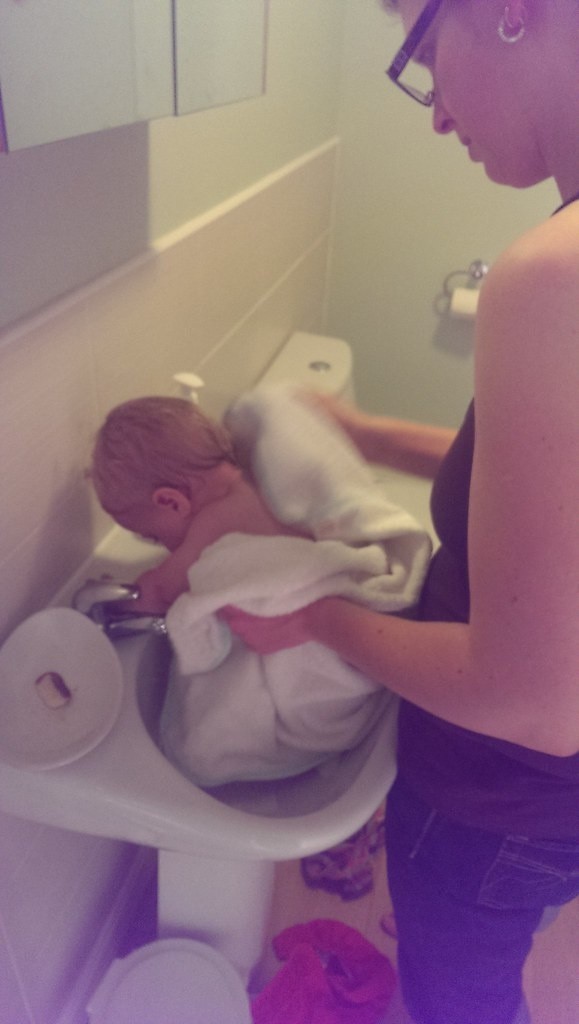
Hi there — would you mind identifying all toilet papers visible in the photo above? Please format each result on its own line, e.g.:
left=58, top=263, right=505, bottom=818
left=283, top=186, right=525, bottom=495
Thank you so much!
left=446, top=288, right=481, bottom=320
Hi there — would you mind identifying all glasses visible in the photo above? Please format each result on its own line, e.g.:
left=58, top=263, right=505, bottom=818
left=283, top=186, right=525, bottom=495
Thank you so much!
left=381, top=1, right=439, bottom=107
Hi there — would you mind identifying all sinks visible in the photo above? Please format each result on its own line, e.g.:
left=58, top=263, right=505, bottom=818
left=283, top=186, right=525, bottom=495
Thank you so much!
left=3, top=523, right=407, bottom=859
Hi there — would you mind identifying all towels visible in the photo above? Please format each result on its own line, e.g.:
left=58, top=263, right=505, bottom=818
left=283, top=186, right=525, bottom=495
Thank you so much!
left=156, top=378, right=434, bottom=785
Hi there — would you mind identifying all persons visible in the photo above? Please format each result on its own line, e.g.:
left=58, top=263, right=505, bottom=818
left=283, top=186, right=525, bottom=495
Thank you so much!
left=217, top=0, right=579, bottom=1024
left=90, top=396, right=290, bottom=618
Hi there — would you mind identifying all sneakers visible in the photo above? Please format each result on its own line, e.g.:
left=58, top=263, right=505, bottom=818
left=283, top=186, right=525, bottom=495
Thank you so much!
left=341, top=815, right=388, bottom=853
left=298, top=851, right=374, bottom=902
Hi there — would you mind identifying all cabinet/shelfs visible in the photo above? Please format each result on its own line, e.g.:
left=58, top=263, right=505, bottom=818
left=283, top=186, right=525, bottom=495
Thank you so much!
left=0, top=0, right=267, bottom=146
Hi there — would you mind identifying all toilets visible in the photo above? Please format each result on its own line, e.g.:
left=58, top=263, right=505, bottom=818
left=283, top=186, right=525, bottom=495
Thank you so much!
left=260, top=330, right=356, bottom=407
